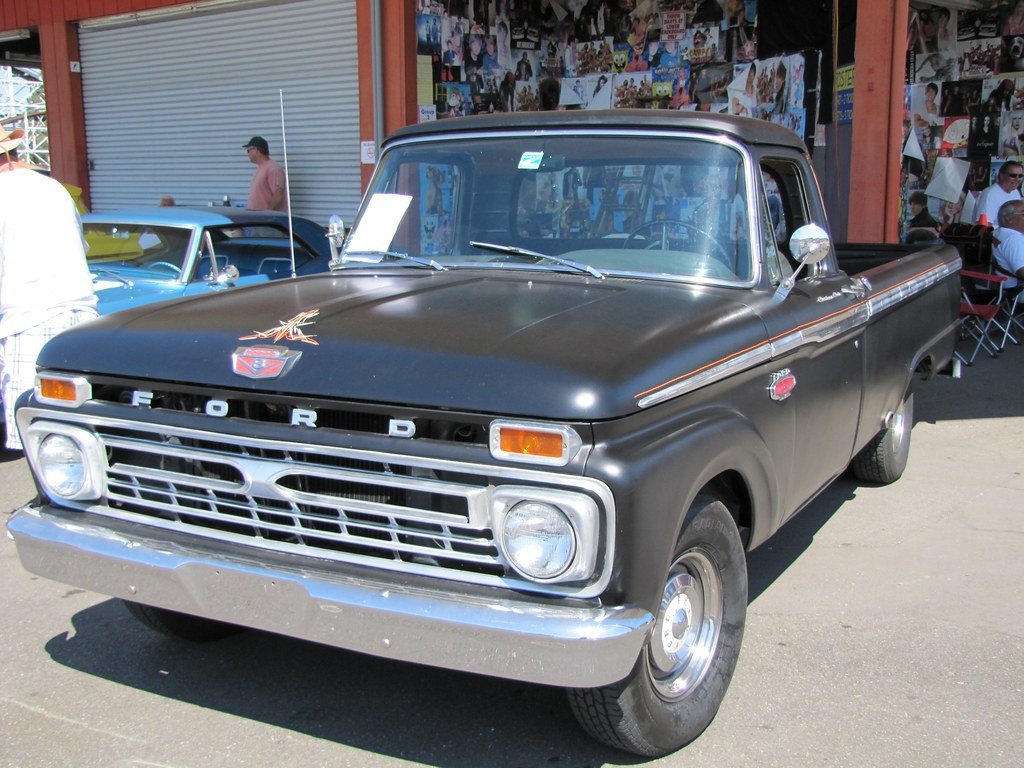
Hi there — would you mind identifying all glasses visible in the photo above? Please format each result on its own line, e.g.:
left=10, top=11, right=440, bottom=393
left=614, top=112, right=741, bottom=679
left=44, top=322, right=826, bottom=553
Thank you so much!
left=1001, top=171, right=1023, bottom=179
left=1006, top=212, right=1024, bottom=216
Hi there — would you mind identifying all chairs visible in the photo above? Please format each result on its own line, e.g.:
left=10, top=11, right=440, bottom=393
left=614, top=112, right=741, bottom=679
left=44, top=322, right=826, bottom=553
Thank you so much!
left=904, top=226, right=1022, bottom=368
left=938, top=222, right=1024, bottom=346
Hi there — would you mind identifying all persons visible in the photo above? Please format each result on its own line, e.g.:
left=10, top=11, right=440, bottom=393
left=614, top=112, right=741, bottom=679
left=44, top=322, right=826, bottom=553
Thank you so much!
left=240, top=136, right=287, bottom=239
left=973, top=161, right=1022, bottom=227
left=992, top=199, right=1024, bottom=305
left=416, top=2, right=1024, bottom=242
left=0, top=119, right=105, bottom=452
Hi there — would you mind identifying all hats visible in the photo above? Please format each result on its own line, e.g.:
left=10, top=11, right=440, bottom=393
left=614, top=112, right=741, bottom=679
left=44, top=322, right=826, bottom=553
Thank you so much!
left=0, top=126, right=24, bottom=156
left=241, top=137, right=270, bottom=156
